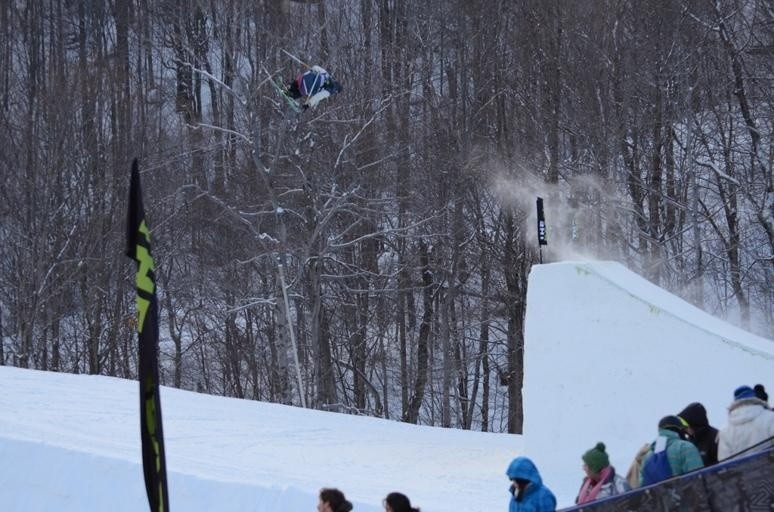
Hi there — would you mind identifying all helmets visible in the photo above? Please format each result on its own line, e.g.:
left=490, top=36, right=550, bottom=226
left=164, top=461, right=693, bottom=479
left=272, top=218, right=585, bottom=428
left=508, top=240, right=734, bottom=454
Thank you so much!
left=659, top=416, right=696, bottom=438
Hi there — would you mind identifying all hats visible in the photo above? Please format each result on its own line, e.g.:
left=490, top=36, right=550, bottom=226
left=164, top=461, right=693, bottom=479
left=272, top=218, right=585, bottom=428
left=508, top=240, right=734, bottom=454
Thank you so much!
left=582, top=443, right=609, bottom=473
left=753, top=384, right=768, bottom=402
left=734, top=386, right=756, bottom=400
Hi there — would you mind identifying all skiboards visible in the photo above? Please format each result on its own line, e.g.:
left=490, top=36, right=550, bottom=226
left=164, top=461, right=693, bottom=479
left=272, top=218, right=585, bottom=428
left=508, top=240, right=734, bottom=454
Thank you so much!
left=262, top=64, right=303, bottom=114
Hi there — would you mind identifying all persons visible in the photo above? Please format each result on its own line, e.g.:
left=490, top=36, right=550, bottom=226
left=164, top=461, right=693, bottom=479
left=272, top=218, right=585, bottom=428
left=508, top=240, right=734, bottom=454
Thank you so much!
left=508, top=457, right=556, bottom=512
left=318, top=489, right=352, bottom=512
left=576, top=385, right=774, bottom=512
left=384, top=493, right=419, bottom=512
